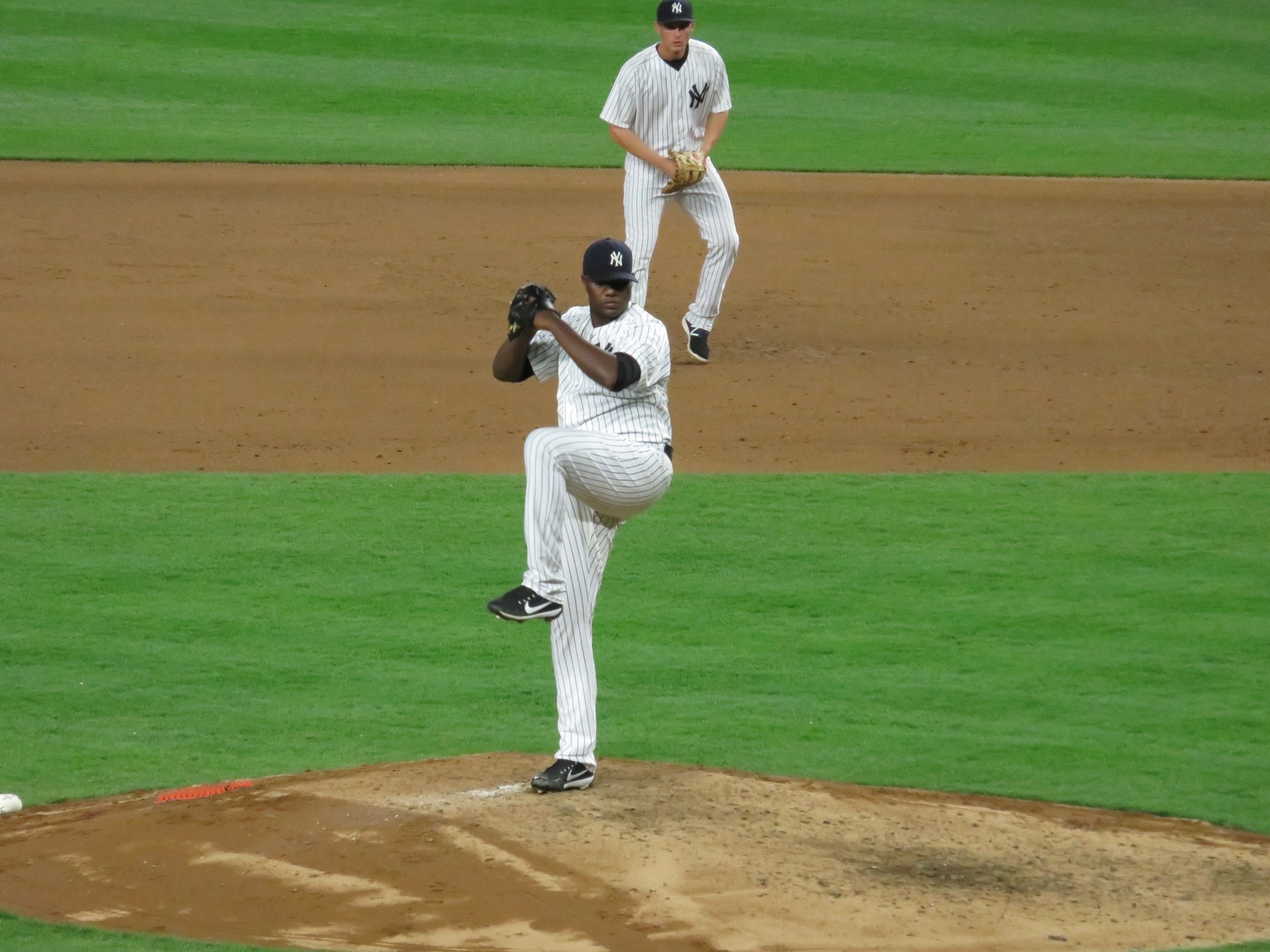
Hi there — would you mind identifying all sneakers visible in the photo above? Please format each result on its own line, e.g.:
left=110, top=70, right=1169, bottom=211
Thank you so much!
left=487, top=586, right=562, bottom=623
left=532, top=760, right=595, bottom=794
left=682, top=315, right=709, bottom=362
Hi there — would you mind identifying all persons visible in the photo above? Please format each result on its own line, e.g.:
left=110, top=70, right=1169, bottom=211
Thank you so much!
left=487, top=238, right=675, bottom=793
left=600, top=0, right=740, bottom=363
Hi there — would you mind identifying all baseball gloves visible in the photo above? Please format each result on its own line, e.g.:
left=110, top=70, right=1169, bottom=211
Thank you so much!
left=661, top=147, right=708, bottom=195
left=507, top=282, right=562, bottom=343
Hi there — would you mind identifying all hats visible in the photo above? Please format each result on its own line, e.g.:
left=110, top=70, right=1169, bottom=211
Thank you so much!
left=657, top=0, right=694, bottom=22
left=584, top=238, right=638, bottom=283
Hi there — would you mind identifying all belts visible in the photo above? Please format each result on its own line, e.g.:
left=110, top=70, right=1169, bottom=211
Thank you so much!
left=663, top=442, right=675, bottom=460
